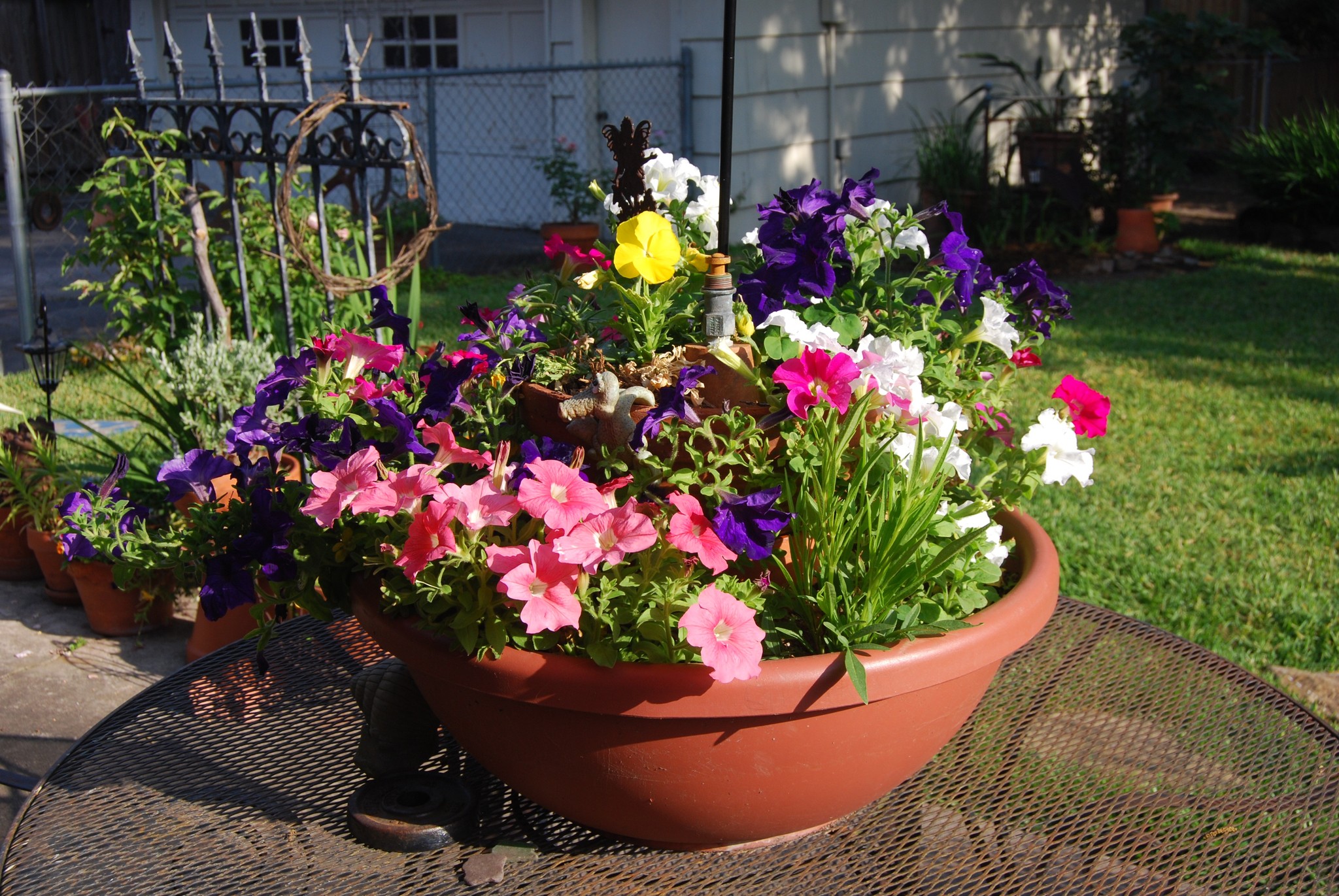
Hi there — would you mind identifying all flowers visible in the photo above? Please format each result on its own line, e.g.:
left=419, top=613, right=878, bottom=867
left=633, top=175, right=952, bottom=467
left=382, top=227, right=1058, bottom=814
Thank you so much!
left=53, top=134, right=1111, bottom=708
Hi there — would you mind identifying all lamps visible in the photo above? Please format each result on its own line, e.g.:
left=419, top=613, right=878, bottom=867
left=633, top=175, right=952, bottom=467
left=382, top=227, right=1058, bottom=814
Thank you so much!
left=16, top=294, right=72, bottom=394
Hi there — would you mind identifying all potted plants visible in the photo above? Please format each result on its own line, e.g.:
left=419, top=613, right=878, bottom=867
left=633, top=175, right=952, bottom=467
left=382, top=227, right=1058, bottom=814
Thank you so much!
left=917, top=50, right=1086, bottom=244
left=0, top=405, right=85, bottom=591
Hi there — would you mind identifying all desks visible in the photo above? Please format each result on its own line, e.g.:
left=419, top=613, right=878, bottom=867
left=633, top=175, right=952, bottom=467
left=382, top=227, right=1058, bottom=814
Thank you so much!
left=0, top=596, right=1339, bottom=896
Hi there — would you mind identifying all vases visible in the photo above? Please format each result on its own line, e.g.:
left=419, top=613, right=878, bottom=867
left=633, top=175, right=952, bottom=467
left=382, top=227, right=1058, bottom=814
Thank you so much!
left=66, top=560, right=176, bottom=636
left=175, top=452, right=302, bottom=529
left=350, top=481, right=1060, bottom=853
left=519, top=346, right=889, bottom=600
left=542, top=223, right=600, bottom=272
